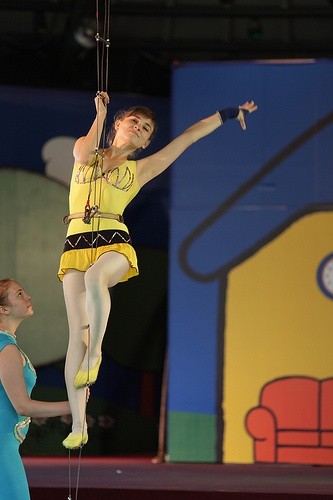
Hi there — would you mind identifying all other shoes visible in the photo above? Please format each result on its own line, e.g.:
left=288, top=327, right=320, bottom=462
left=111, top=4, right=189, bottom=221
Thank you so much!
left=73, top=353, right=102, bottom=389
left=62, top=432, right=89, bottom=449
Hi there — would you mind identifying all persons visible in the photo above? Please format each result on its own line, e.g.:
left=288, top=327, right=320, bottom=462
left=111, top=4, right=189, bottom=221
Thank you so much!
left=60, top=90, right=257, bottom=449
left=0, top=279, right=91, bottom=500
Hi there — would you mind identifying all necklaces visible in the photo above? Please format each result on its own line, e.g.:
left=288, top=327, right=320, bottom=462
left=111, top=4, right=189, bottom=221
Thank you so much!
left=0, top=329, right=17, bottom=340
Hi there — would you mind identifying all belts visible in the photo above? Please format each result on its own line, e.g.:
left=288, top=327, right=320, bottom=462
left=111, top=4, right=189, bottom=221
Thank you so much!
left=68, top=212, right=120, bottom=221
